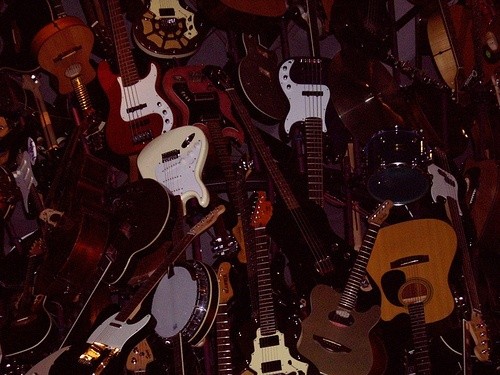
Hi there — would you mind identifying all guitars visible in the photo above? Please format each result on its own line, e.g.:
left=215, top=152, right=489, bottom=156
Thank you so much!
left=212, top=262, right=240, bottom=375
left=296, top=199, right=388, bottom=375
left=1, top=237, right=53, bottom=356
left=278, top=56, right=333, bottom=137
left=204, top=61, right=339, bottom=275
left=136, top=125, right=210, bottom=217
left=208, top=1, right=289, bottom=31
left=458, top=82, right=500, bottom=256
left=131, top=1, right=201, bottom=59
left=443, top=197, right=500, bottom=375
left=366, top=218, right=458, bottom=375
left=366, top=128, right=430, bottom=221
left=57, top=179, right=170, bottom=346
left=96, top=1, right=176, bottom=152
left=162, top=65, right=245, bottom=203
left=151, top=258, right=220, bottom=375
left=36, top=18, right=103, bottom=153
left=425, top=0, right=500, bottom=159
left=51, top=204, right=225, bottom=375
left=233, top=191, right=310, bottom=375
left=0, top=65, right=62, bottom=117
left=26, top=107, right=107, bottom=294
left=331, top=0, right=395, bottom=57
left=236, top=22, right=289, bottom=124
